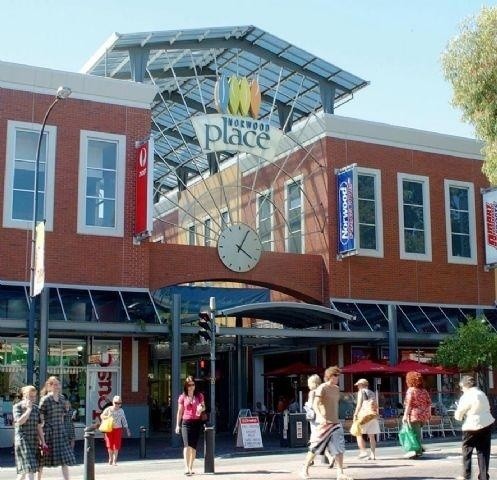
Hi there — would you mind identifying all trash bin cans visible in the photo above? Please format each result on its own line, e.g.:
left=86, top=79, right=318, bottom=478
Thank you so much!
left=288, top=413, right=309, bottom=448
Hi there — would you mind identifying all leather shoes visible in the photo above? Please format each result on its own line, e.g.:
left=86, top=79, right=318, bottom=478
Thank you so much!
left=184, top=471, right=195, bottom=476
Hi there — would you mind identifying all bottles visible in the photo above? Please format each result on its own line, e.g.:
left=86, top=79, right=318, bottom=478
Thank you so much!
left=196, top=404, right=203, bottom=416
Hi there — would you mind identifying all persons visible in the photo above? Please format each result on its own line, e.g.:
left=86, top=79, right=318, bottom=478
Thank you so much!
left=304, top=374, right=335, bottom=469
left=453, top=375, right=496, bottom=480
left=255, top=402, right=269, bottom=414
left=352, top=378, right=379, bottom=460
left=404, top=371, right=433, bottom=459
left=12, top=385, right=48, bottom=480
left=296, top=365, right=355, bottom=480
left=99, top=395, right=131, bottom=466
left=277, top=395, right=298, bottom=413
left=39, top=377, right=76, bottom=480
left=175, top=376, right=207, bottom=475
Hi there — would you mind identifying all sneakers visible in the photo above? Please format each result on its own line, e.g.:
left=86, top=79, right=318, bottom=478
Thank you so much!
left=407, top=446, right=425, bottom=460
left=356, top=452, right=376, bottom=462
left=309, top=455, right=337, bottom=469
left=335, top=474, right=353, bottom=480
left=299, top=464, right=311, bottom=479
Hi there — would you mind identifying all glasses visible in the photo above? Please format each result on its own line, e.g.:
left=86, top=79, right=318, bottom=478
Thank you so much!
left=332, top=373, right=339, bottom=377
left=114, top=402, right=121, bottom=405
left=187, top=384, right=194, bottom=387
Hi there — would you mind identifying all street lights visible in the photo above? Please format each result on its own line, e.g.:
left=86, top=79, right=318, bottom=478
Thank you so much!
left=24, top=83, right=73, bottom=411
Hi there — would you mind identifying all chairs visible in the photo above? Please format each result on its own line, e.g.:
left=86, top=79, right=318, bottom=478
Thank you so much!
left=251, top=407, right=457, bottom=443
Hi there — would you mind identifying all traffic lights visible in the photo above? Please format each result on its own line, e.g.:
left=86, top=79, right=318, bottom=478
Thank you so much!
left=197, top=309, right=214, bottom=347
left=197, top=356, right=210, bottom=379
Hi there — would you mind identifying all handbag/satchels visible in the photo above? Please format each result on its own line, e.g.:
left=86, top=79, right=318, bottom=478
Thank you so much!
left=305, top=408, right=316, bottom=421
left=200, top=411, right=209, bottom=424
left=397, top=421, right=422, bottom=454
left=98, top=408, right=113, bottom=433
left=357, top=389, right=378, bottom=425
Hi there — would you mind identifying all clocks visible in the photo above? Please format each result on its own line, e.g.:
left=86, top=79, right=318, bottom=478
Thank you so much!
left=216, top=223, right=263, bottom=273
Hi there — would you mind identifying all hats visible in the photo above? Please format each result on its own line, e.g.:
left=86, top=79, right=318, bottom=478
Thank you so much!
left=353, top=378, right=368, bottom=387
